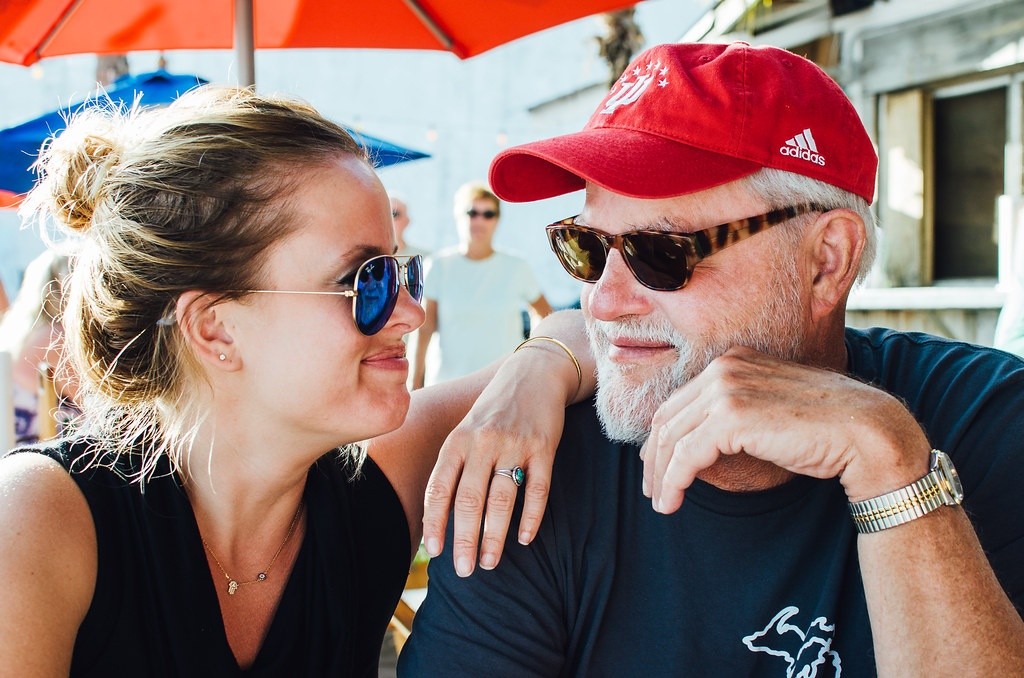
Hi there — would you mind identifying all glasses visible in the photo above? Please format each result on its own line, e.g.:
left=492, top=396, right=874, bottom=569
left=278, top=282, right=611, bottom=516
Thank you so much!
left=544, top=203, right=842, bottom=291
left=217, top=255, right=425, bottom=336
left=468, top=210, right=497, bottom=219
left=393, top=211, right=406, bottom=217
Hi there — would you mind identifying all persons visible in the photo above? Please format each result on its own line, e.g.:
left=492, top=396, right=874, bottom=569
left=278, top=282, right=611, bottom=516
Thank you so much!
left=389, top=197, right=431, bottom=264
left=1, top=84, right=597, bottom=678
left=394, top=44, right=1024, bottom=678
left=405, top=181, right=555, bottom=392
left=1, top=245, right=83, bottom=406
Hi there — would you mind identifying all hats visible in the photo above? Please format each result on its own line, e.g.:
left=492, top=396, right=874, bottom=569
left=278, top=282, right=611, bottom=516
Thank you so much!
left=488, top=42, right=878, bottom=205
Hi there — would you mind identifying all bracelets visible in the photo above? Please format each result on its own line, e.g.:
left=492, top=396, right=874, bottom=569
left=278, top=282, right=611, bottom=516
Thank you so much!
left=514, top=335, right=583, bottom=408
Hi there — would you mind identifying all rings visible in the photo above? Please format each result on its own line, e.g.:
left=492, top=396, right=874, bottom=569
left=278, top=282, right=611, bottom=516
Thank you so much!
left=494, top=465, right=525, bottom=488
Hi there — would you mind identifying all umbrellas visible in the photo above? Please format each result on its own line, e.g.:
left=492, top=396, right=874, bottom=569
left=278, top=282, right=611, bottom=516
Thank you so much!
left=1, top=58, right=431, bottom=209
left=0, top=0, right=645, bottom=94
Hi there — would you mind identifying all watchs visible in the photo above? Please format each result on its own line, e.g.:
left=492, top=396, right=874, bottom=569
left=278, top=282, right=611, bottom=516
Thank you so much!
left=847, top=449, right=964, bottom=534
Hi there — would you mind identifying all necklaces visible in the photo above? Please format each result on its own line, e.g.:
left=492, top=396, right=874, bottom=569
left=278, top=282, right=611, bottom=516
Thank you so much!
left=200, top=487, right=308, bottom=595
